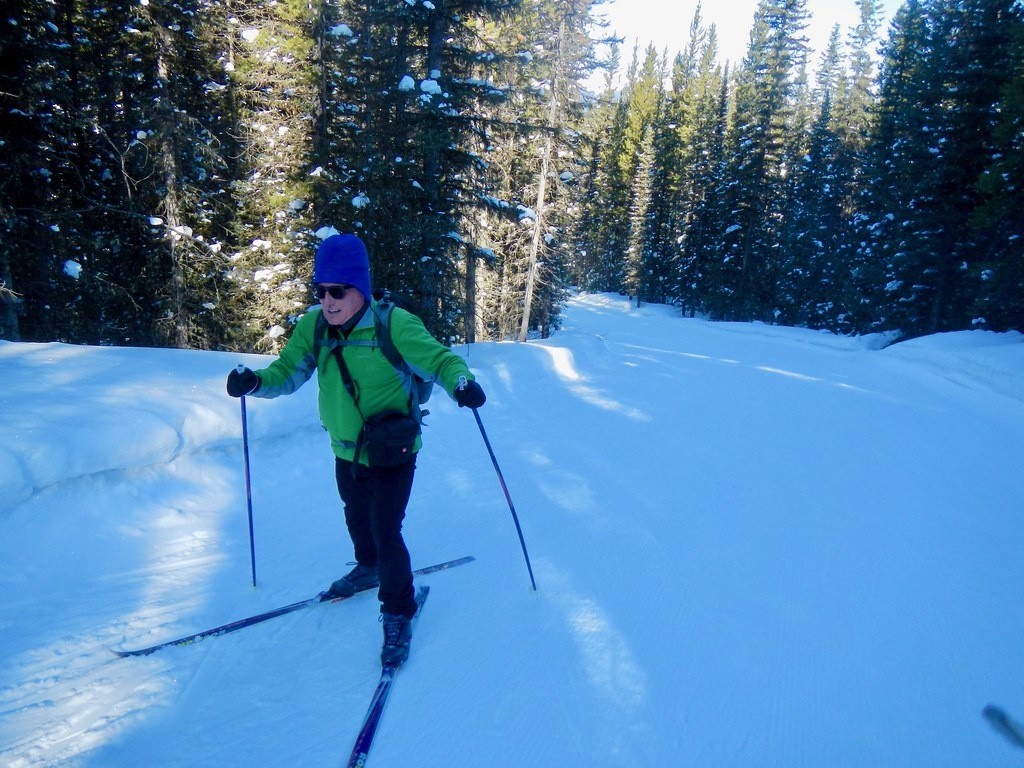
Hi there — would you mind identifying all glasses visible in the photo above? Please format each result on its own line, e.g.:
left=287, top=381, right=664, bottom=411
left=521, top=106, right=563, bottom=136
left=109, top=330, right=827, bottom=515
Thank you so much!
left=312, top=285, right=354, bottom=299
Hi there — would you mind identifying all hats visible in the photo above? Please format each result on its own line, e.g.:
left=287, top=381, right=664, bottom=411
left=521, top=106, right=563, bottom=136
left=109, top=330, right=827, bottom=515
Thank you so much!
left=312, top=234, right=372, bottom=301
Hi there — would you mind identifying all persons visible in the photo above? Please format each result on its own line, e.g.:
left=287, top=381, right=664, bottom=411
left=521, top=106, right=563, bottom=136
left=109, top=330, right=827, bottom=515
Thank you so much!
left=227, top=236, right=487, bottom=666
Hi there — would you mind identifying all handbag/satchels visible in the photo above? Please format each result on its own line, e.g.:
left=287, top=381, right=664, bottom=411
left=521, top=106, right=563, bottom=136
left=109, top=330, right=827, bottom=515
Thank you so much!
left=363, top=409, right=420, bottom=465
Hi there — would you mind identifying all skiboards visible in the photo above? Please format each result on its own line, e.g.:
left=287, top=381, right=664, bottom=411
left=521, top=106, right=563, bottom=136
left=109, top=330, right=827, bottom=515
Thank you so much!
left=100, top=555, right=477, bottom=768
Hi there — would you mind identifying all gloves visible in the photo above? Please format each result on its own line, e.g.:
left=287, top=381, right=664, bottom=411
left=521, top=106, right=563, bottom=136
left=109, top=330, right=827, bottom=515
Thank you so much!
left=453, top=380, right=486, bottom=408
left=226, top=367, right=259, bottom=398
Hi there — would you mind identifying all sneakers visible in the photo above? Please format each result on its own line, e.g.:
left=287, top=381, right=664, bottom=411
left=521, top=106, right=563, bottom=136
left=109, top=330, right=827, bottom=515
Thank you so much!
left=329, top=561, right=380, bottom=598
left=378, top=613, right=413, bottom=671
